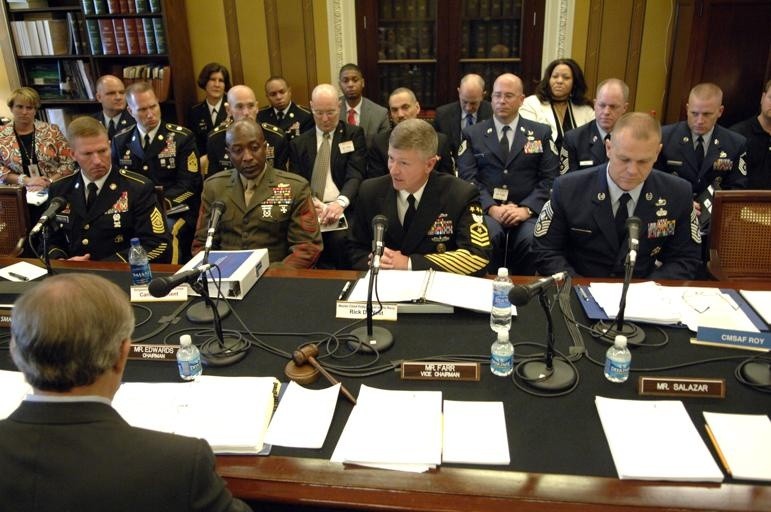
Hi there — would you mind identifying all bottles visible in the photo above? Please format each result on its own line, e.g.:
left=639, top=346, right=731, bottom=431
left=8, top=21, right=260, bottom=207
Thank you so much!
left=490, top=328, right=515, bottom=377
left=127, top=237, right=154, bottom=287
left=604, top=335, right=632, bottom=383
left=491, top=268, right=514, bottom=313
left=174, top=333, right=204, bottom=383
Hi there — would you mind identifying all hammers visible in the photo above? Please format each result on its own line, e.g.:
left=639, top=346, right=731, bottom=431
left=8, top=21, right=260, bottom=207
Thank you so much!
left=292, top=344, right=356, bottom=406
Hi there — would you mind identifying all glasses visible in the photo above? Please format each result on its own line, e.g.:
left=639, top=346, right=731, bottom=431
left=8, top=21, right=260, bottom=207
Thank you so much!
left=491, top=92, right=521, bottom=103
left=311, top=107, right=338, bottom=120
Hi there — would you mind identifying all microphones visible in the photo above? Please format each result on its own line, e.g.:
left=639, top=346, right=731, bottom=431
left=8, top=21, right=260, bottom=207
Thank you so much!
left=370, top=212, right=390, bottom=277
left=147, top=263, right=213, bottom=297
left=30, top=194, right=67, bottom=239
left=508, top=269, right=570, bottom=308
left=623, top=214, right=644, bottom=271
left=203, top=198, right=227, bottom=251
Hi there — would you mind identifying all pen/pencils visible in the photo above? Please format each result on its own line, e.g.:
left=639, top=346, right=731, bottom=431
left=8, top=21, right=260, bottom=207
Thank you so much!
left=9, top=272, right=29, bottom=281
left=338, top=281, right=351, bottom=300
left=577, top=283, right=590, bottom=302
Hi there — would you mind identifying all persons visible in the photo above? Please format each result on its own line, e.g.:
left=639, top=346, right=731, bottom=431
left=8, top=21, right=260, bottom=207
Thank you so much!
left=0, top=56, right=771, bottom=278
left=1, top=271, right=257, bottom=512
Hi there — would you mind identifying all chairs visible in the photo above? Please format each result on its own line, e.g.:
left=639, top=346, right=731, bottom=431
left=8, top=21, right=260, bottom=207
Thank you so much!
left=708, top=189, right=771, bottom=283
left=1, top=185, right=32, bottom=257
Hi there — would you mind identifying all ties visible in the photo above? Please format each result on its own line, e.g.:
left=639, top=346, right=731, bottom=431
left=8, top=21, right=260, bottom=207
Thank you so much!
left=614, top=192, right=632, bottom=249
left=500, top=126, right=510, bottom=159
left=277, top=111, right=284, bottom=123
left=694, top=136, right=704, bottom=169
left=402, top=194, right=416, bottom=236
left=86, top=183, right=98, bottom=209
left=143, top=134, right=150, bottom=151
left=245, top=179, right=255, bottom=207
left=465, top=113, right=473, bottom=127
left=310, top=133, right=330, bottom=201
left=108, top=119, right=115, bottom=139
left=347, top=109, right=356, bottom=126
left=604, top=134, right=611, bottom=149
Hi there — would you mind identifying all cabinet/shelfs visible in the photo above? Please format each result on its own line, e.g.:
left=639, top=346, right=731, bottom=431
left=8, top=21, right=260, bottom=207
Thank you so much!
left=356, top=1, right=547, bottom=126
left=0, top=0, right=200, bottom=130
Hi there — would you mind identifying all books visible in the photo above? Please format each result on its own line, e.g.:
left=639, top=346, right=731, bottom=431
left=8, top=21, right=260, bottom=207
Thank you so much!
left=376, top=1, right=523, bottom=108
left=174, top=247, right=270, bottom=300
left=348, top=269, right=519, bottom=318
left=6, top=0, right=174, bottom=136
left=0, top=369, right=512, bottom=476
left=589, top=281, right=761, bottom=333
